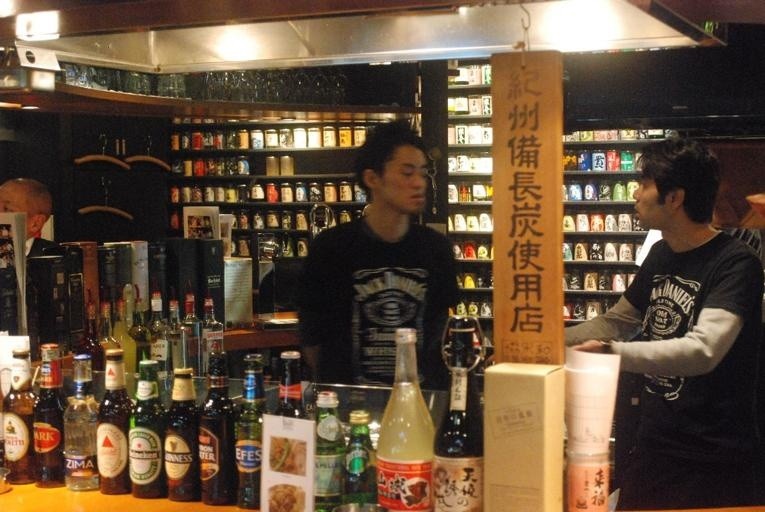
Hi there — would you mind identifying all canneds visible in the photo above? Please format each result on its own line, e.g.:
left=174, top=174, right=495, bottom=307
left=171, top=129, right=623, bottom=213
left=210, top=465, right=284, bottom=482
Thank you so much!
left=448, top=66, right=681, bottom=322
left=173, top=125, right=417, bottom=259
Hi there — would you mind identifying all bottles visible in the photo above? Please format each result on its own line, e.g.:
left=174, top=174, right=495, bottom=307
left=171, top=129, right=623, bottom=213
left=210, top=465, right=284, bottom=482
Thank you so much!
left=238, top=355, right=270, bottom=508
left=66, top=352, right=100, bottom=492
left=129, top=358, right=167, bottom=499
left=31, top=342, right=67, bottom=490
left=83, top=289, right=229, bottom=397
left=166, top=363, right=201, bottom=503
left=197, top=350, right=236, bottom=506
left=4, top=346, right=36, bottom=486
left=100, top=347, right=131, bottom=496
left=275, top=317, right=614, bottom=509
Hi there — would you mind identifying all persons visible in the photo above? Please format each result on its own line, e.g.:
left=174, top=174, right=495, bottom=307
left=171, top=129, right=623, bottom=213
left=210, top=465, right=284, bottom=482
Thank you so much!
left=0, top=177, right=82, bottom=358
left=298, top=123, right=462, bottom=421
left=564, top=137, right=764, bottom=510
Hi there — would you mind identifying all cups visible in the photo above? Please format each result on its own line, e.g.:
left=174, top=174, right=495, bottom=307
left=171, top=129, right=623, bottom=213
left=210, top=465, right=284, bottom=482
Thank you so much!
left=447, top=213, right=494, bottom=288
left=562, top=179, right=647, bottom=294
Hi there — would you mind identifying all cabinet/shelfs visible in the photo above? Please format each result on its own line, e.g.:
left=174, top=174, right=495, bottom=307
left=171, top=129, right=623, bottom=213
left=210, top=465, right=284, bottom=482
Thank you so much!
left=563, top=139, right=666, bottom=325
left=447, top=85, right=493, bottom=319
left=172, top=121, right=389, bottom=261
